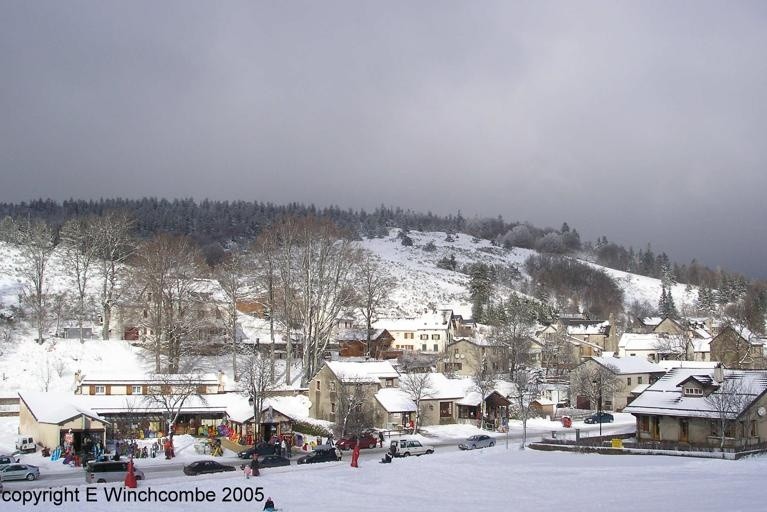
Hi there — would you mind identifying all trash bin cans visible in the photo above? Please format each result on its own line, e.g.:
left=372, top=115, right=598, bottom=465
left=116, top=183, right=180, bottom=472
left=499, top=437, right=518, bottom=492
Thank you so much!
left=552, top=431, right=557, bottom=438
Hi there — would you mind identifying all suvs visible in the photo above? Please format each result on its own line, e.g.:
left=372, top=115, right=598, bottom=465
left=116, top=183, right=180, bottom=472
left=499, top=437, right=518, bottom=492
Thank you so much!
left=390, top=440, right=434, bottom=458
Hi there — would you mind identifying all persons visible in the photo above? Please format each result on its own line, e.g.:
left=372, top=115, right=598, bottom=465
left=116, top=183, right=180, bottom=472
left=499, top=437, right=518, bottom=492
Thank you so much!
left=476, top=407, right=506, bottom=433
left=263, top=497, right=278, bottom=512
left=561, top=403, right=572, bottom=427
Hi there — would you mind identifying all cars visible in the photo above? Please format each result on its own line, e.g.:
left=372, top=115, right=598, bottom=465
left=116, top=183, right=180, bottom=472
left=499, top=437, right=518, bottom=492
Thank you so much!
left=584, top=412, right=614, bottom=424
left=0, top=463, right=40, bottom=482
left=183, top=460, right=236, bottom=476
left=458, top=434, right=496, bottom=450
left=335, top=432, right=378, bottom=450
left=238, top=442, right=338, bottom=471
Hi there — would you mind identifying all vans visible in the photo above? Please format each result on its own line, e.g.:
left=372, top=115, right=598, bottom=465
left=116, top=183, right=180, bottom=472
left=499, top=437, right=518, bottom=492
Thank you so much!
left=86, top=461, right=145, bottom=485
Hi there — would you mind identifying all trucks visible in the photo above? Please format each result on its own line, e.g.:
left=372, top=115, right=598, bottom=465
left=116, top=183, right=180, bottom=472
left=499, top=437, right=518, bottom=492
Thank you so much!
left=15, top=435, right=36, bottom=453
